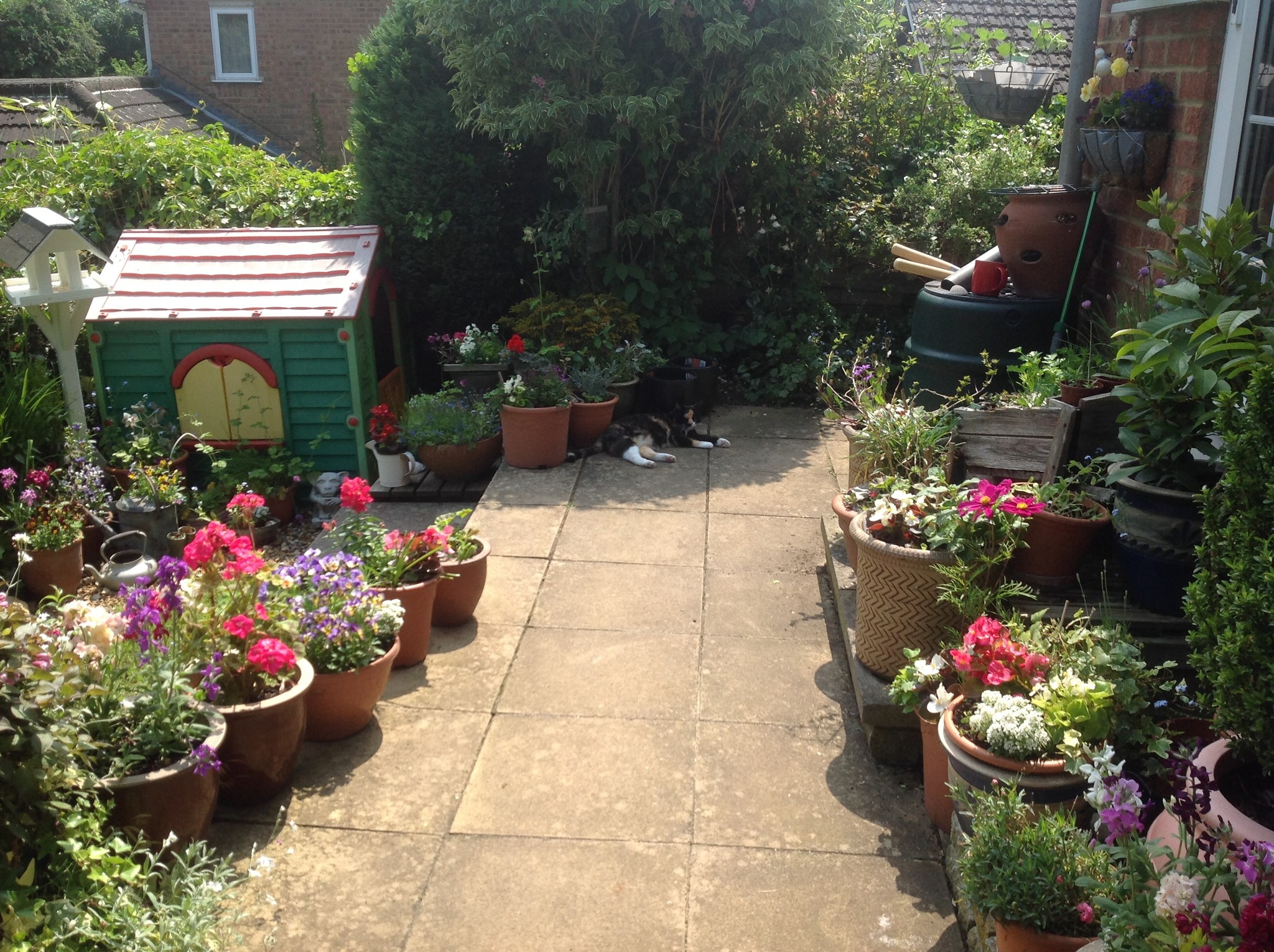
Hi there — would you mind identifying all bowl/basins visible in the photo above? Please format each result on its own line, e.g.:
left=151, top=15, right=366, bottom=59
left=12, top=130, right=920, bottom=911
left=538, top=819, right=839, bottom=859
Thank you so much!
left=644, top=355, right=719, bottom=421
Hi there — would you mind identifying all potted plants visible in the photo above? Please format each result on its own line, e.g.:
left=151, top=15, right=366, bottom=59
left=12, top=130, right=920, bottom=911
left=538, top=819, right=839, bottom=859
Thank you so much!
left=813, top=332, right=996, bottom=491
left=398, top=381, right=503, bottom=483
left=493, top=225, right=669, bottom=447
left=1005, top=455, right=1111, bottom=589
left=1058, top=188, right=1274, bottom=613
left=1143, top=357, right=1274, bottom=920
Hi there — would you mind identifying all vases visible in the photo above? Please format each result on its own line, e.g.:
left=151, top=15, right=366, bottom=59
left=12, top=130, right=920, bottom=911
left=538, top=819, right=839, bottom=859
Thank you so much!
left=995, top=192, right=1106, bottom=299
left=16, top=447, right=494, bottom=866
left=364, top=438, right=416, bottom=489
left=831, top=464, right=1006, bottom=683
left=995, top=916, right=1100, bottom=952
left=642, top=355, right=720, bottom=418
left=1077, top=129, right=1172, bottom=192
left=498, top=396, right=573, bottom=469
left=914, top=696, right=954, bottom=835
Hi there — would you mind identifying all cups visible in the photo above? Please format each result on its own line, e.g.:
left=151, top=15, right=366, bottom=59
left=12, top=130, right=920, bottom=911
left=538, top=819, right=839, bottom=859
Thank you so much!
left=971, top=260, right=1008, bottom=298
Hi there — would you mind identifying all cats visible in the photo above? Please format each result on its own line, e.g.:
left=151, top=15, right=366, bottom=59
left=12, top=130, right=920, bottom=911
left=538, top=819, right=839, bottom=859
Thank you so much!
left=566, top=399, right=731, bottom=469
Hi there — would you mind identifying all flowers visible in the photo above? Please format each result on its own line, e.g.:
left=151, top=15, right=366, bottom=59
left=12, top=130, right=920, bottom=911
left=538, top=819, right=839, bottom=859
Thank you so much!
left=1079, top=57, right=1177, bottom=130
left=0, top=395, right=456, bottom=779
left=884, top=617, right=1274, bottom=951
left=369, top=322, right=570, bottom=452
left=366, top=404, right=407, bottom=452
left=865, top=475, right=1050, bottom=554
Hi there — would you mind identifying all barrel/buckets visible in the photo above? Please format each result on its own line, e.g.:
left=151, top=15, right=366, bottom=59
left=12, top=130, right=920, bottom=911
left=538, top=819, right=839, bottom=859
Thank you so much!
left=900, top=278, right=1080, bottom=411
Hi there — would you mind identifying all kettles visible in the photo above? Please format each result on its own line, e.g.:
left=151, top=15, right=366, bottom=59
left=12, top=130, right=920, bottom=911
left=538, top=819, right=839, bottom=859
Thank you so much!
left=80, top=529, right=159, bottom=592
